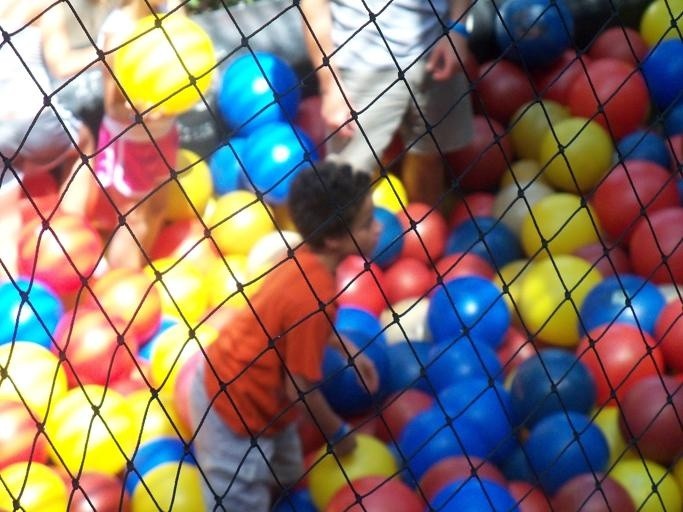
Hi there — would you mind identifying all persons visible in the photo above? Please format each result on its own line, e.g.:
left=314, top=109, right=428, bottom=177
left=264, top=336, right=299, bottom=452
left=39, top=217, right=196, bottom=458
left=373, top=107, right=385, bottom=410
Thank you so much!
left=301, top=0, right=473, bottom=215
left=192, top=161, right=379, bottom=512
left=0, top=0, right=191, bottom=268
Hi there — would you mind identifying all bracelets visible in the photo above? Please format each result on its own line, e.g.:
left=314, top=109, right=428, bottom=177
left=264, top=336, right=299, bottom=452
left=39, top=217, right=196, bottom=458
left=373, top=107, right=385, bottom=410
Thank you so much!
left=332, top=422, right=349, bottom=442
left=447, top=19, right=467, bottom=36
